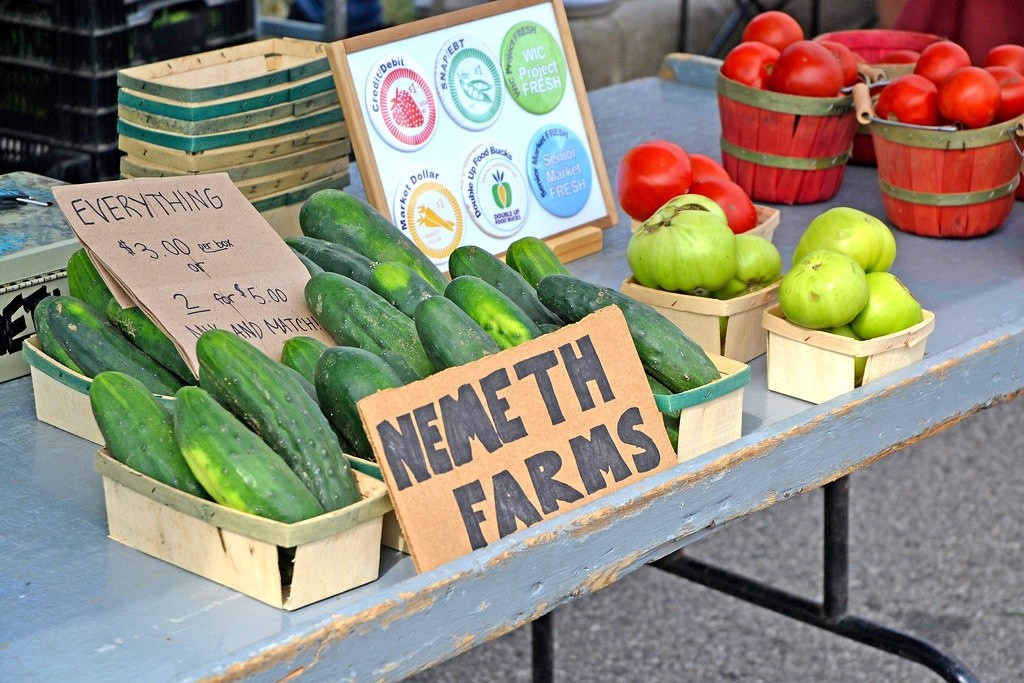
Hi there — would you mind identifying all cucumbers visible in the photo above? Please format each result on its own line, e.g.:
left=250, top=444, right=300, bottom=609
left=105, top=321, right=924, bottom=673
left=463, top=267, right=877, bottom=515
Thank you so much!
left=33, top=188, right=723, bottom=584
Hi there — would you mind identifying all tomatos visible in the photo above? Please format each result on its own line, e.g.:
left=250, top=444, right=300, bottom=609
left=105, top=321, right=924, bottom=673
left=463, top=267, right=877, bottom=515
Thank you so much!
left=724, top=12, right=1024, bottom=133
left=617, top=139, right=923, bottom=388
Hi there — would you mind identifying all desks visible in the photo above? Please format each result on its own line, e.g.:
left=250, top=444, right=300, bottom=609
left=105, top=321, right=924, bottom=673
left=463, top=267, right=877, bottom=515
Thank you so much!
left=0, top=54, right=1024, bottom=683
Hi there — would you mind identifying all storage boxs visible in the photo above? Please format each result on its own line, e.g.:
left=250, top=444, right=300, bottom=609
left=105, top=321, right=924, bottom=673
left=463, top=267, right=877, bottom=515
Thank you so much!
left=0, top=0, right=261, bottom=186
